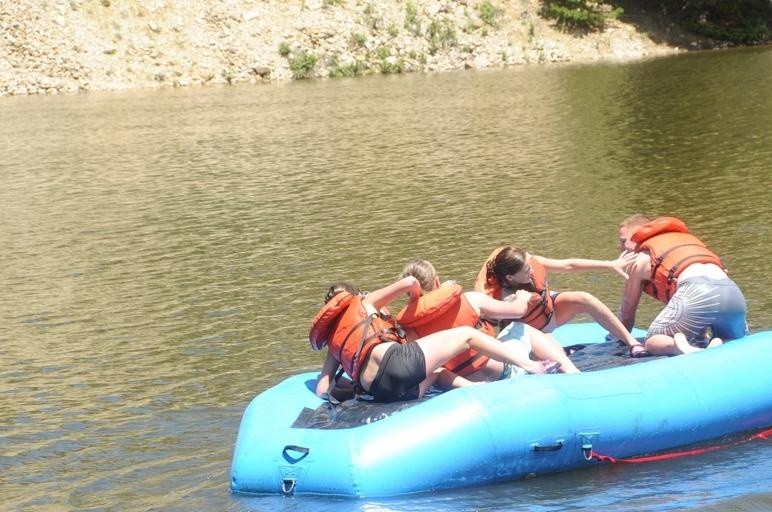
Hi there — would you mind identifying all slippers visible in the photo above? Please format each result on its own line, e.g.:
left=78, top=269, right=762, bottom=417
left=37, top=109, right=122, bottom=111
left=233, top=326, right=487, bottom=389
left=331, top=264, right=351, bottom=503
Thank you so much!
left=538, top=359, right=562, bottom=375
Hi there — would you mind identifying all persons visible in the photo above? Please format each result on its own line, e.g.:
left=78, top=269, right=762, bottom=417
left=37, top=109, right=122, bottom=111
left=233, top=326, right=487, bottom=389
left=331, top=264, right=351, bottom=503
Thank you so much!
left=394, top=260, right=582, bottom=380
left=475, top=244, right=648, bottom=375
left=316, top=276, right=562, bottom=402
left=614, top=214, right=743, bottom=354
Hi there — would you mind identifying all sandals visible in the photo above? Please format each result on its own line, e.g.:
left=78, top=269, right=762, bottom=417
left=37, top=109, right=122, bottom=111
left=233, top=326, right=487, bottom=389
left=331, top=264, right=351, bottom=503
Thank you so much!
left=629, top=344, right=650, bottom=358
